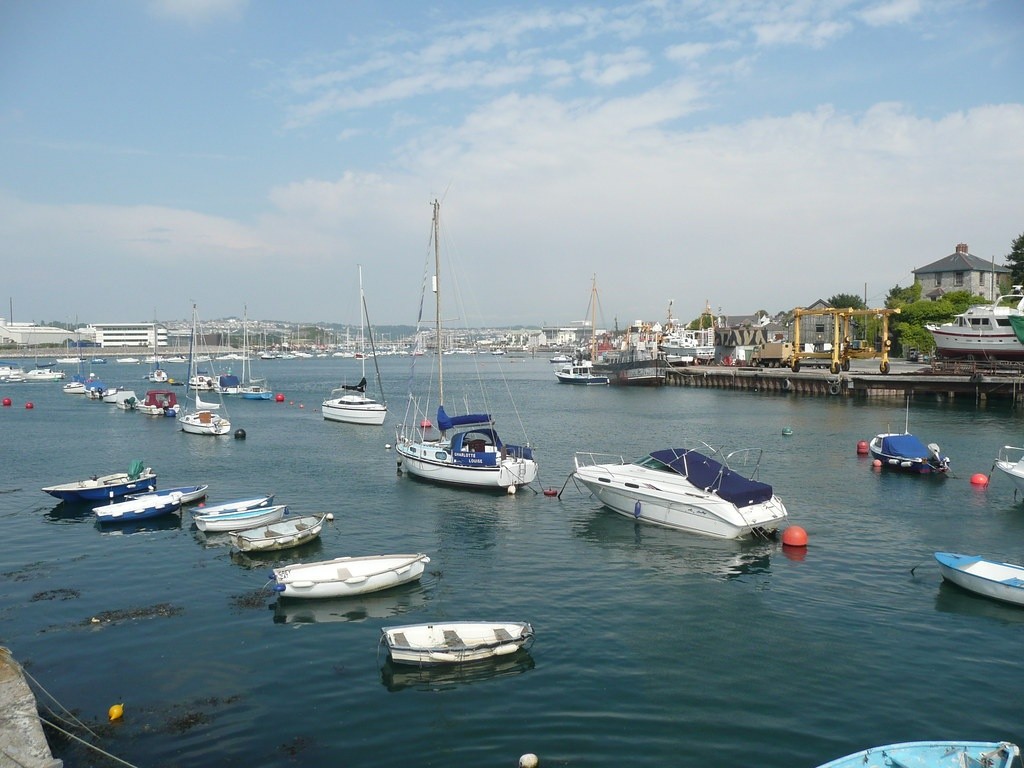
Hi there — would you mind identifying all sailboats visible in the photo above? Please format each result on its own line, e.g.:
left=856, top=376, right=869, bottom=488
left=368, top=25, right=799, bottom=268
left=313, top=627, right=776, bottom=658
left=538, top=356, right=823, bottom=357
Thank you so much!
left=321, top=262, right=388, bottom=426
left=391, top=200, right=537, bottom=492
left=273, top=326, right=510, bottom=360
left=0, top=304, right=275, bottom=435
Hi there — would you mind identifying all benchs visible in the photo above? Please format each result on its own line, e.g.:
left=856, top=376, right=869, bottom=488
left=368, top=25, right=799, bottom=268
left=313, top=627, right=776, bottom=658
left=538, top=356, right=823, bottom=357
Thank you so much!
left=339, top=570, right=352, bottom=579
left=394, top=633, right=411, bottom=648
left=1001, top=577, right=1024, bottom=587
left=295, top=523, right=312, bottom=530
left=265, top=530, right=284, bottom=538
left=494, top=628, right=513, bottom=639
left=445, top=630, right=467, bottom=649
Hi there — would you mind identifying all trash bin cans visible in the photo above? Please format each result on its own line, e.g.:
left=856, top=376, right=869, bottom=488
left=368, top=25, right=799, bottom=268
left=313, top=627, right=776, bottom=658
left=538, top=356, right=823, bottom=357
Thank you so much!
left=917, top=354, right=923, bottom=363
left=693, top=358, right=699, bottom=366
left=735, top=359, right=741, bottom=367
left=923, top=354, right=928, bottom=362
left=740, top=360, right=747, bottom=367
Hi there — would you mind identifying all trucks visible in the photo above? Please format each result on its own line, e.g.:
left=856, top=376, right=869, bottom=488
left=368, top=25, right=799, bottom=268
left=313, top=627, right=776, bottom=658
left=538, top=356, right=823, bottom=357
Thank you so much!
left=750, top=342, right=793, bottom=369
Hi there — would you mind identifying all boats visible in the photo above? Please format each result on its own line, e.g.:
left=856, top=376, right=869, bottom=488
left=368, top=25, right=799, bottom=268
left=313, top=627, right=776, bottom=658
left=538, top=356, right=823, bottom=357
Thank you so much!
left=548, top=271, right=715, bottom=385
left=382, top=649, right=531, bottom=695
left=41, top=466, right=209, bottom=521
left=925, top=291, right=1024, bottom=363
left=230, top=539, right=322, bottom=571
left=188, top=492, right=331, bottom=551
left=193, top=526, right=236, bottom=549
left=270, top=549, right=430, bottom=599
left=96, top=514, right=180, bottom=535
left=814, top=740, right=1019, bottom=768
left=381, top=622, right=532, bottom=667
left=268, top=582, right=438, bottom=624
left=867, top=429, right=1024, bottom=504
left=935, top=550, right=1023, bottom=607
left=571, top=438, right=792, bottom=543
